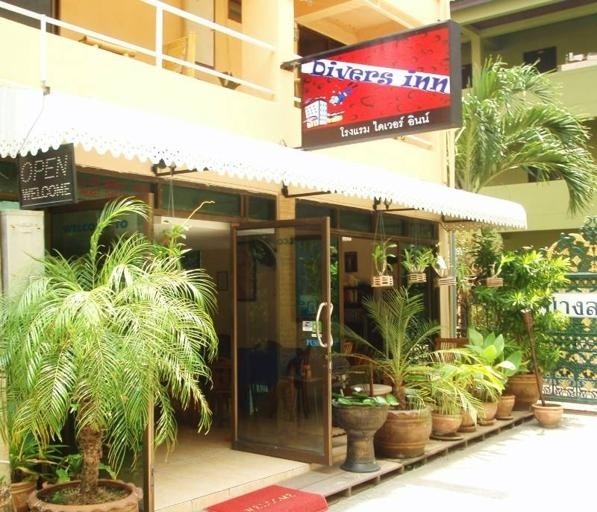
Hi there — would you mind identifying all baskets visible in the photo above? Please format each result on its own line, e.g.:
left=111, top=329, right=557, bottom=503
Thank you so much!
left=483, top=277, right=504, bottom=287
left=406, top=273, right=427, bottom=284
left=433, top=277, right=456, bottom=286
left=371, top=275, right=394, bottom=287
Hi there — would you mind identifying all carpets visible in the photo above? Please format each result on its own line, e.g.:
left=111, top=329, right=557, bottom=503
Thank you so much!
left=203, top=484, right=328, bottom=512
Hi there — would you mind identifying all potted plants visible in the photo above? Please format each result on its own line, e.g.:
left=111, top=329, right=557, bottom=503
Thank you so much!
left=429, top=254, right=458, bottom=289
left=402, top=245, right=433, bottom=284
left=484, top=252, right=516, bottom=288
left=453, top=257, right=483, bottom=288
left=468, top=240, right=568, bottom=408
left=462, top=331, right=504, bottom=427
left=332, top=394, right=392, bottom=471
left=412, top=356, right=462, bottom=441
left=0, top=196, right=220, bottom=512
left=489, top=335, right=518, bottom=419
left=368, top=241, right=397, bottom=290
left=449, top=353, right=483, bottom=433
left=367, top=290, right=435, bottom=460
